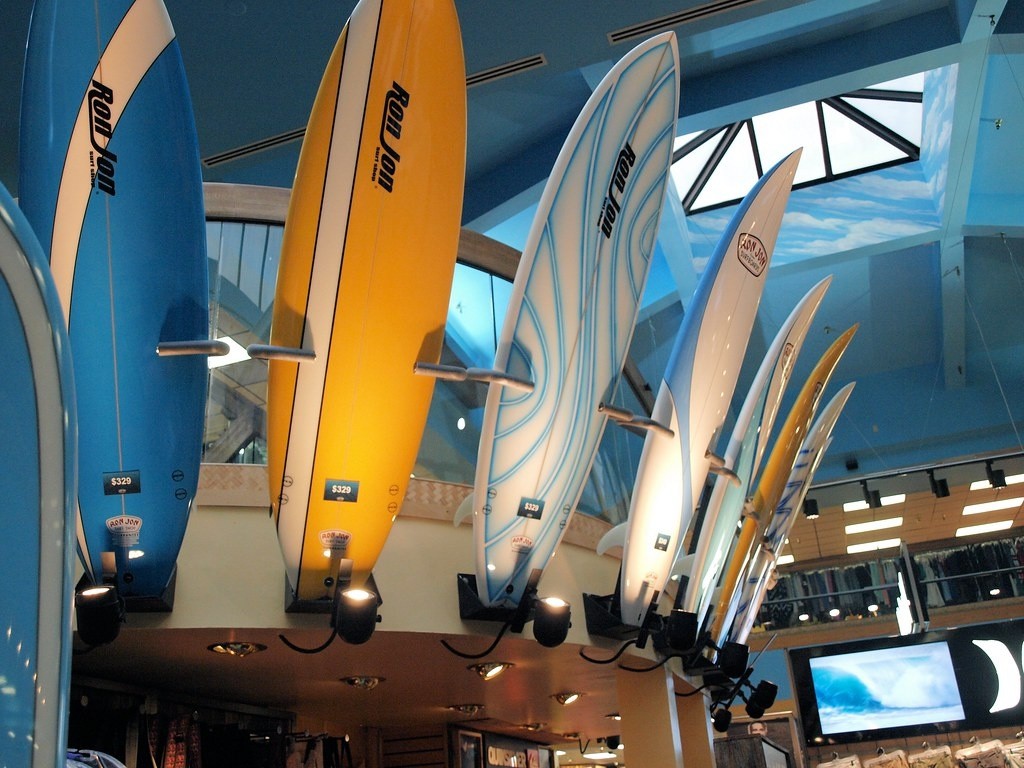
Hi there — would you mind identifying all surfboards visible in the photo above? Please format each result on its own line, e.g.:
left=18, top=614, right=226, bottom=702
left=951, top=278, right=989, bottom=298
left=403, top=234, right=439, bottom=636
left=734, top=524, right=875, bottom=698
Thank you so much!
left=2, top=181, right=86, bottom=768
left=266, top=1, right=470, bottom=617
left=15, top=1, right=211, bottom=603
left=601, top=144, right=806, bottom=624
left=675, top=273, right=862, bottom=676
left=451, top=28, right=687, bottom=612
